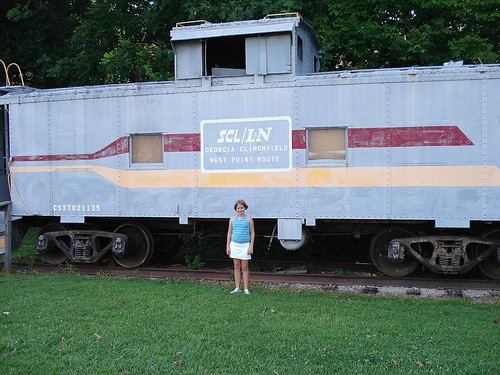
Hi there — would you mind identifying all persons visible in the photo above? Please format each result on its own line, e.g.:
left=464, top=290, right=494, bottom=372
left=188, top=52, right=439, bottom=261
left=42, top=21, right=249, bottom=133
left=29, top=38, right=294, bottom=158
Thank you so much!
left=226, top=200, right=256, bottom=296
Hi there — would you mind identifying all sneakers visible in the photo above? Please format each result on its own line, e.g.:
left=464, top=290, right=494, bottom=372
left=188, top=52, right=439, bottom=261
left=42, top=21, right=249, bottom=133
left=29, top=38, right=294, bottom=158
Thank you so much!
left=230, top=288, right=242, bottom=294
left=244, top=289, right=250, bottom=294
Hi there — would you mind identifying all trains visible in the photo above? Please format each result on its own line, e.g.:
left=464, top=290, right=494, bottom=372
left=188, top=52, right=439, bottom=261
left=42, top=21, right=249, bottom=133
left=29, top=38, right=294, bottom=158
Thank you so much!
left=0, top=11, right=500, bottom=283
